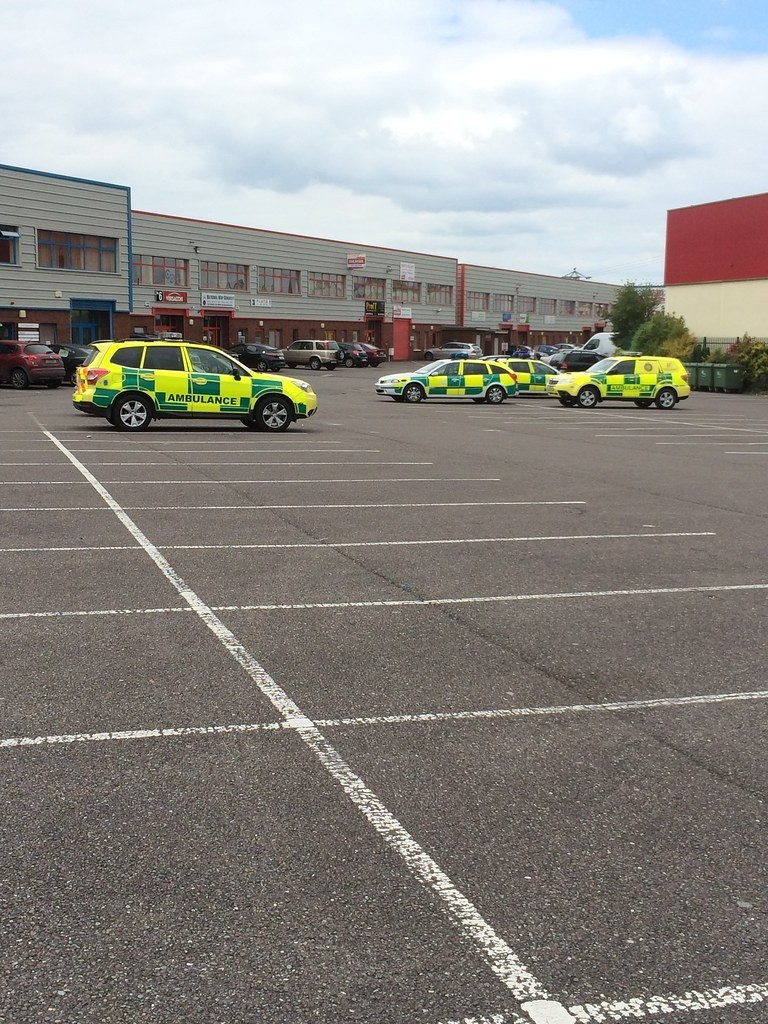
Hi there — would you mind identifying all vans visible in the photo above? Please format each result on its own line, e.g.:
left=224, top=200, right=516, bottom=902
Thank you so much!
left=580, top=332, right=629, bottom=356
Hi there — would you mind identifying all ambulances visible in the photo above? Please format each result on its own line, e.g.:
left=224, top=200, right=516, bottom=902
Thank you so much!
left=483, top=355, right=561, bottom=397
left=375, top=353, right=520, bottom=405
left=546, top=352, right=691, bottom=410
left=72, top=336, right=318, bottom=432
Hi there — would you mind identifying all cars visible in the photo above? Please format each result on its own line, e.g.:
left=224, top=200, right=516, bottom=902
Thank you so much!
left=0, top=340, right=66, bottom=390
left=336, top=343, right=369, bottom=369
left=503, top=343, right=584, bottom=366
left=44, top=343, right=96, bottom=386
left=424, top=341, right=484, bottom=361
left=346, top=341, right=386, bottom=368
left=226, top=342, right=286, bottom=373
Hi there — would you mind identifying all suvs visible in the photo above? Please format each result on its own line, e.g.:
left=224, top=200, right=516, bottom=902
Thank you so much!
left=280, top=340, right=345, bottom=371
left=549, top=349, right=609, bottom=374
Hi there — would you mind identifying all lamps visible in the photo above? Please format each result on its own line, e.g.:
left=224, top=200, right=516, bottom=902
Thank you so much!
left=193, top=246, right=200, bottom=253
left=412, top=325, right=416, bottom=330
left=189, top=319, right=194, bottom=326
left=321, top=323, right=324, bottom=328
left=259, top=321, right=264, bottom=326
left=386, top=266, right=392, bottom=274
left=430, top=325, right=434, bottom=331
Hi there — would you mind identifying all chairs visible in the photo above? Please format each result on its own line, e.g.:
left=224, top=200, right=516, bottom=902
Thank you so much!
left=169, top=350, right=207, bottom=373
